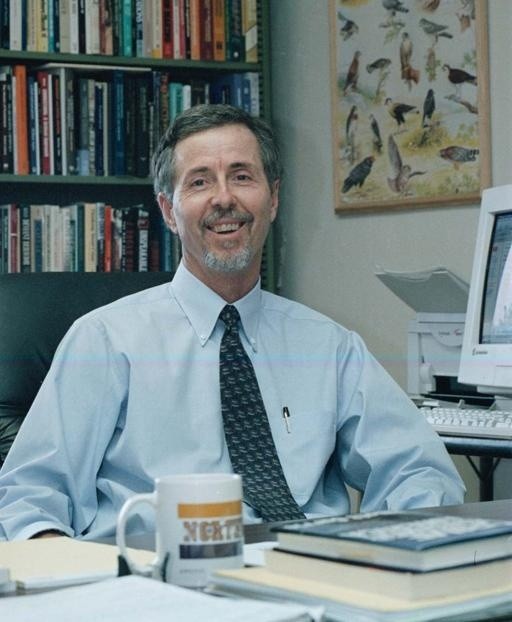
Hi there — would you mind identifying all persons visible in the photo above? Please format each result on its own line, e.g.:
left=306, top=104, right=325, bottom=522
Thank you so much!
left=2, top=103, right=468, bottom=547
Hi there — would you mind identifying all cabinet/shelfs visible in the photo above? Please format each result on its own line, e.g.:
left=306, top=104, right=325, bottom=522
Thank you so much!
left=0, top=0, right=278, bottom=295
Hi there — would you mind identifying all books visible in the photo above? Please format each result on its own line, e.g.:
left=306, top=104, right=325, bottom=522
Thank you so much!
left=1, top=63, right=263, bottom=178
left=205, top=510, right=510, bottom=620
left=2, top=536, right=119, bottom=594
left=2, top=202, right=183, bottom=271
left=2, top=2, right=262, bottom=64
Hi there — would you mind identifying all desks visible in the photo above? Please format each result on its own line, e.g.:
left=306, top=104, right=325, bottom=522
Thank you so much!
left=86, top=441, right=512, bottom=552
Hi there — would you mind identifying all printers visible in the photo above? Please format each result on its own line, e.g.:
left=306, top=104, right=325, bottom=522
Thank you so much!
left=373, top=266, right=499, bottom=408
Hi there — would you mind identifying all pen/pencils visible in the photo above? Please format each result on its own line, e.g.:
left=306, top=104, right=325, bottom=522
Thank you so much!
left=283, top=406, right=293, bottom=434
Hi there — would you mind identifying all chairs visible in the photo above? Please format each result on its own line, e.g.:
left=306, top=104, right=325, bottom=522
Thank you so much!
left=0, top=270, right=173, bottom=467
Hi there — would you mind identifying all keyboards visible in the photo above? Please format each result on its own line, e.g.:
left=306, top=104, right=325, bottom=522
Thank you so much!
left=421, top=406, right=511, bottom=440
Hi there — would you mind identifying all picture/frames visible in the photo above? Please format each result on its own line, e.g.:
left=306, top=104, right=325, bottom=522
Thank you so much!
left=328, top=0, right=489, bottom=215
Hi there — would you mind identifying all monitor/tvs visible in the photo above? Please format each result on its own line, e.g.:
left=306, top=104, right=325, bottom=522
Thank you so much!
left=457, top=185, right=512, bottom=394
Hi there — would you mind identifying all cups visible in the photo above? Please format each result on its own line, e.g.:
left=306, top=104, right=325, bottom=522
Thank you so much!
left=116, top=471, right=247, bottom=588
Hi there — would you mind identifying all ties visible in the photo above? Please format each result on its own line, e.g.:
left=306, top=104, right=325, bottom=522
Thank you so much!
left=218, top=306, right=308, bottom=522
